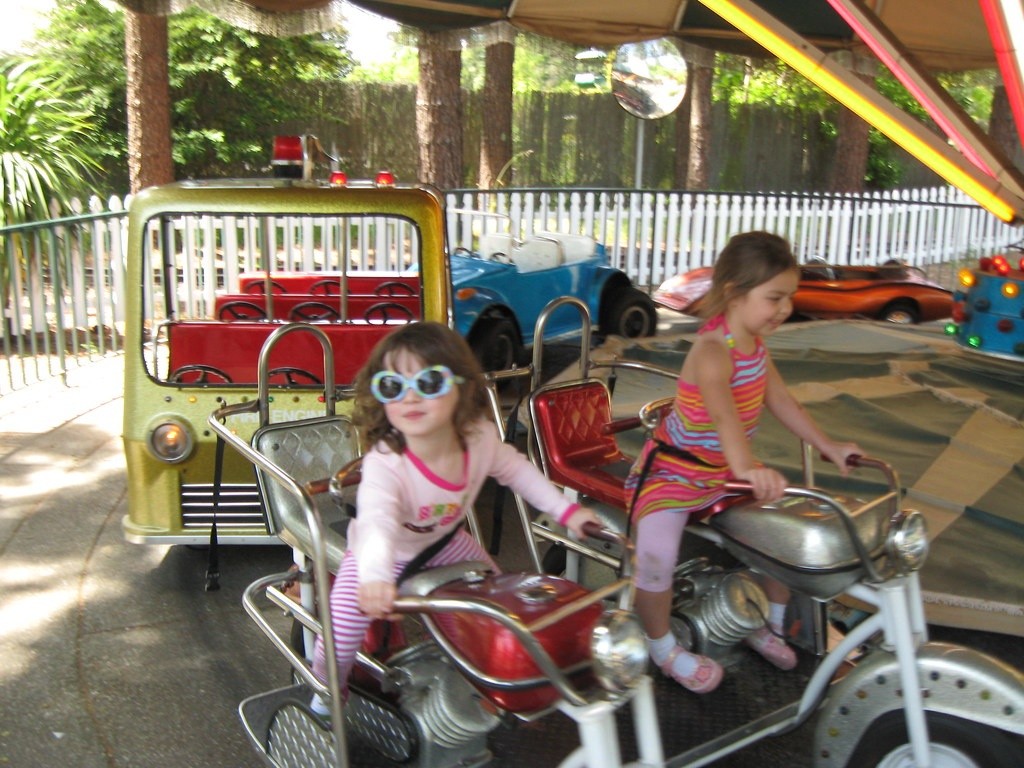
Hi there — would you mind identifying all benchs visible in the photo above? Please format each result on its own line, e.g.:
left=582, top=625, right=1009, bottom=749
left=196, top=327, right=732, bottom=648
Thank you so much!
left=213, top=293, right=420, bottom=320
left=238, top=273, right=420, bottom=295
left=152, top=321, right=420, bottom=386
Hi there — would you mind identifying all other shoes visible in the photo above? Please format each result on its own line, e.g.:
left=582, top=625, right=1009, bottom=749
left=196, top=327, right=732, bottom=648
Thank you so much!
left=743, top=623, right=796, bottom=670
left=657, top=644, right=724, bottom=694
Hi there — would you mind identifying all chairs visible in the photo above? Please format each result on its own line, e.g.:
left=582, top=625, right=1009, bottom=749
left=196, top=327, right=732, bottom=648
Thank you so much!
left=517, top=235, right=562, bottom=271
left=252, top=413, right=361, bottom=578
left=529, top=378, right=634, bottom=511
left=479, top=232, right=517, bottom=263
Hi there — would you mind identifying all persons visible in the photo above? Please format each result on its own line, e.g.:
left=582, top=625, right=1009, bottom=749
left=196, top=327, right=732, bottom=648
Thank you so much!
left=309, top=318, right=623, bottom=723
left=625, top=230, right=871, bottom=695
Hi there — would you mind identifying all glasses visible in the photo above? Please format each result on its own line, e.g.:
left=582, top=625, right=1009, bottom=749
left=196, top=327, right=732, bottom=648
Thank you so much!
left=369, top=364, right=467, bottom=403
